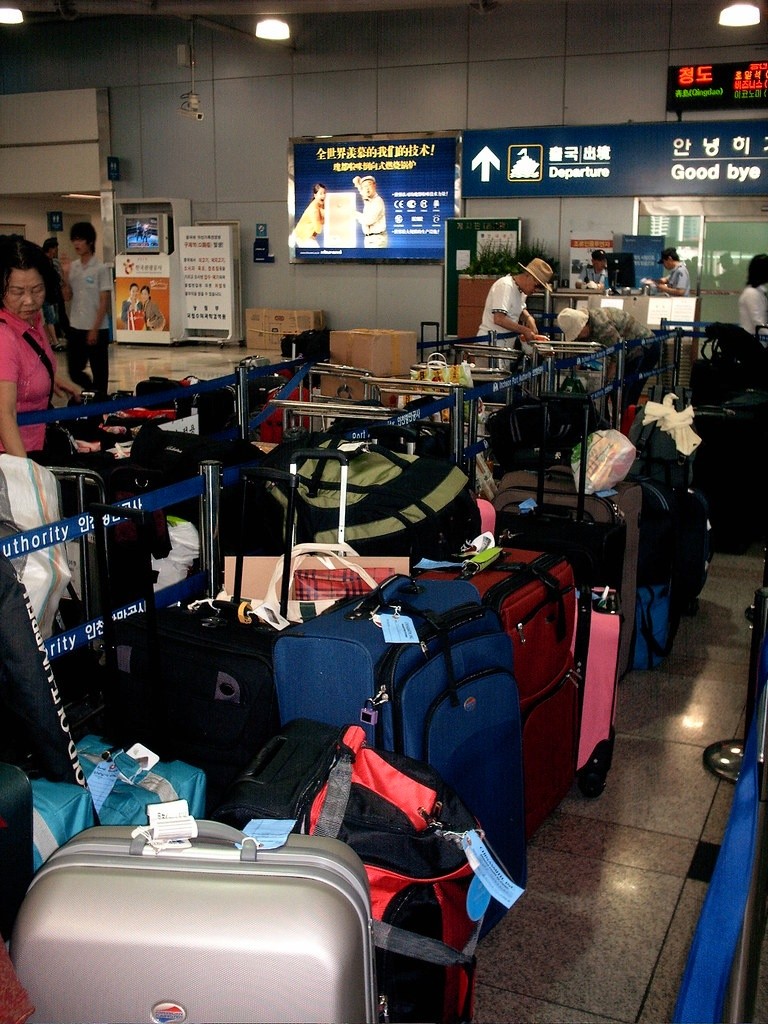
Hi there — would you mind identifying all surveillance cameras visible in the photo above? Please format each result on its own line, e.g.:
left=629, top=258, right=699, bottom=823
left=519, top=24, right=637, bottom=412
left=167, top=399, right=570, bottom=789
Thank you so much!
left=177, top=109, right=203, bottom=121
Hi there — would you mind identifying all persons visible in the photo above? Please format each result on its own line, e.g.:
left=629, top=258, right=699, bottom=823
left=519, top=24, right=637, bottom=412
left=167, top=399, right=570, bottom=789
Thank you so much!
left=474, top=258, right=554, bottom=370
left=42, top=237, right=66, bottom=352
left=58, top=222, right=112, bottom=395
left=121, top=283, right=143, bottom=330
left=353, top=175, right=388, bottom=248
left=140, top=285, right=165, bottom=331
left=739, top=253, right=768, bottom=347
left=575, top=249, right=608, bottom=290
left=136, top=220, right=150, bottom=242
left=0, top=234, right=81, bottom=458
left=654, top=247, right=690, bottom=298
left=294, top=182, right=326, bottom=248
left=557, top=308, right=660, bottom=429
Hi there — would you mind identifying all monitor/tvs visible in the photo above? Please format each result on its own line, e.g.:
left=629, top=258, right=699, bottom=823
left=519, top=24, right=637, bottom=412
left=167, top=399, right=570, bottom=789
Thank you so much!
left=122, top=214, right=169, bottom=256
left=605, top=252, right=635, bottom=297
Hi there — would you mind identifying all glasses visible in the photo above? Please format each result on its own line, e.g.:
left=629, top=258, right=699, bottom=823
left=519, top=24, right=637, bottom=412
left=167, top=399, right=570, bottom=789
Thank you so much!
left=533, top=280, right=543, bottom=290
left=71, top=237, right=84, bottom=242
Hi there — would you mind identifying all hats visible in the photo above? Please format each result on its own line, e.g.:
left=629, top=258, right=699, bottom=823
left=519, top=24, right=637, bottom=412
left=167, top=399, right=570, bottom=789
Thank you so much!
left=557, top=307, right=589, bottom=342
left=592, top=249, right=608, bottom=260
left=518, top=258, right=554, bottom=291
left=657, top=248, right=679, bottom=264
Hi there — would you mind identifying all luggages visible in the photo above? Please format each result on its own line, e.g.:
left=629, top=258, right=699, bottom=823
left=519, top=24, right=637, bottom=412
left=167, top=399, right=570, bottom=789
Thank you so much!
left=0, top=326, right=711, bottom=1024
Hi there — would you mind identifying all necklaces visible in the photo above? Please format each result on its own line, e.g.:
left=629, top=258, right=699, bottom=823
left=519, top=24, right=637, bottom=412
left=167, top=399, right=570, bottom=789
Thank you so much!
left=512, top=276, right=522, bottom=293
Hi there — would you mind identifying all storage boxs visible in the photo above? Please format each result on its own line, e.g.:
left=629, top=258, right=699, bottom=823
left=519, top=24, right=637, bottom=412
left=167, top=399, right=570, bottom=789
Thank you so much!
left=225, top=555, right=409, bottom=625
left=246, top=307, right=325, bottom=350
left=330, top=328, right=418, bottom=376
left=321, top=374, right=414, bottom=409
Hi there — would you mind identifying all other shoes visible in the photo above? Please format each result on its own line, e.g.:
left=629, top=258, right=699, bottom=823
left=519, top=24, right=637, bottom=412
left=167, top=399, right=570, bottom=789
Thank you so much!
left=51, top=343, right=68, bottom=352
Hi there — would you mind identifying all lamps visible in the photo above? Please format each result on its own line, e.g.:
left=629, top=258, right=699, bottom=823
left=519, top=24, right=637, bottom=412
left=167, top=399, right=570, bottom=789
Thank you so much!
left=719, top=0, right=761, bottom=27
left=0, top=0, right=24, bottom=24
left=255, top=14, right=290, bottom=40
